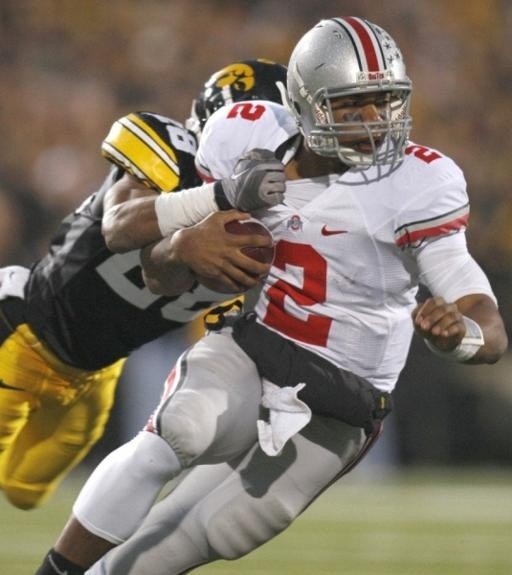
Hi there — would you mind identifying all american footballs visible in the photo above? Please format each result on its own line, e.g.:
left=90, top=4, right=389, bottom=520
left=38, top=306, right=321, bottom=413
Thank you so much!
left=197, top=219, right=274, bottom=293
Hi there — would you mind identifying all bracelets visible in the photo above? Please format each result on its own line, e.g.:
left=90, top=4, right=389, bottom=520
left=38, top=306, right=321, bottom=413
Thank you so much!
left=425, top=315, right=485, bottom=365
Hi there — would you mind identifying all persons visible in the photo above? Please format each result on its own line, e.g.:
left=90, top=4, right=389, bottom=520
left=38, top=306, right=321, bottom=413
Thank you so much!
left=32, top=15, right=508, bottom=574
left=0, top=58, right=306, bottom=512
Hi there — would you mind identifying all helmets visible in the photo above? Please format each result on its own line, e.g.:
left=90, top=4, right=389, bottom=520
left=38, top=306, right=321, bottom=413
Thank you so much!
left=186, top=58, right=288, bottom=148
left=288, top=14, right=414, bottom=171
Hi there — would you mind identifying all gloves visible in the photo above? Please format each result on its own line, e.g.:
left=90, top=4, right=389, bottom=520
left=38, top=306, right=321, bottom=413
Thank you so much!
left=221, top=148, right=286, bottom=212
